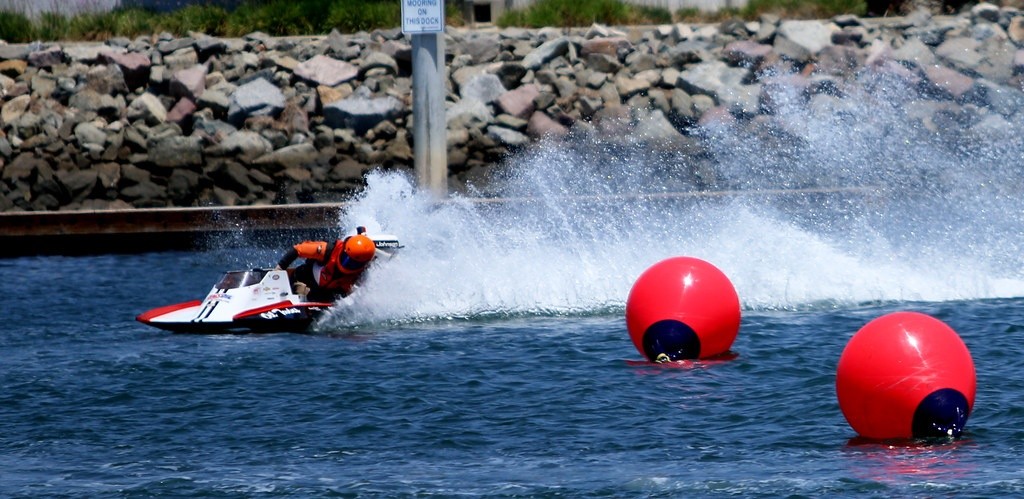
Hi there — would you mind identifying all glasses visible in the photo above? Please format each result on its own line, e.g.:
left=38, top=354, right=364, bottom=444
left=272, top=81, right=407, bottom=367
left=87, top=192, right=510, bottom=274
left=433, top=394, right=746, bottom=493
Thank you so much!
left=339, top=250, right=368, bottom=270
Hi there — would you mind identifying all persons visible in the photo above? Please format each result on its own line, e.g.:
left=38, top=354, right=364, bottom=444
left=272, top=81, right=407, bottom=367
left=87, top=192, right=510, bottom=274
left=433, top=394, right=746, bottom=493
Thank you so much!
left=275, top=235, right=375, bottom=304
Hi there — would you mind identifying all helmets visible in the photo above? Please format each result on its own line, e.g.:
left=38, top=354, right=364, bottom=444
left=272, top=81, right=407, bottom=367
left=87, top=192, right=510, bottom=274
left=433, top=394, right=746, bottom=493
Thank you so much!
left=337, top=235, right=375, bottom=273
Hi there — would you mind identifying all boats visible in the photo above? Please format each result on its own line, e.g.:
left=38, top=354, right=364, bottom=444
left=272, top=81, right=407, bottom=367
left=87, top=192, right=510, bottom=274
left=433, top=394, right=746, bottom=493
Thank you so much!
left=137, top=226, right=405, bottom=332
